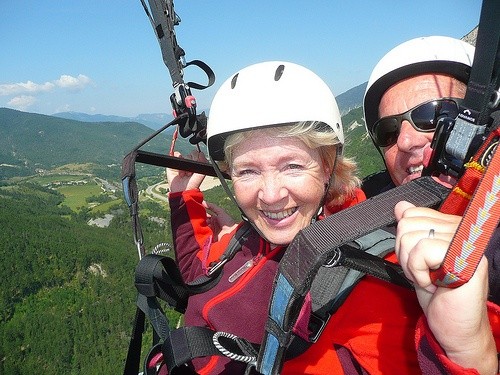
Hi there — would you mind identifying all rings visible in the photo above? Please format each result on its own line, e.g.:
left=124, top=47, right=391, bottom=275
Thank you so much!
left=429, top=229, right=435, bottom=239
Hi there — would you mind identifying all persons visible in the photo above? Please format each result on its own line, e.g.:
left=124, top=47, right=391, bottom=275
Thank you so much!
left=201, top=35, right=500, bottom=306
left=156, top=61, right=500, bottom=375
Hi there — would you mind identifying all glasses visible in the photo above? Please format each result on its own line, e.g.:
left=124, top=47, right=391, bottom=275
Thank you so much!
left=372, top=97, right=465, bottom=147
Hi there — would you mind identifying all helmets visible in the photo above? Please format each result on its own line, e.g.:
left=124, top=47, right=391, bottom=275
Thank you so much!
left=363, top=35, right=476, bottom=138
left=206, top=60, right=344, bottom=163
